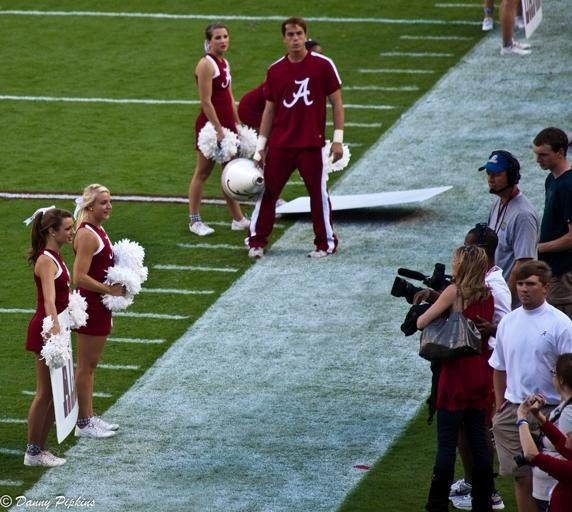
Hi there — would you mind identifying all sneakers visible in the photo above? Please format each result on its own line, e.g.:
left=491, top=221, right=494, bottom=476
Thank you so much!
left=275, top=198, right=287, bottom=219
left=514, top=15, right=524, bottom=29
left=232, top=217, right=251, bottom=230
left=189, top=221, right=215, bottom=236
left=501, top=40, right=531, bottom=55
left=482, top=18, right=494, bottom=31
left=307, top=249, right=326, bottom=258
left=24, top=450, right=67, bottom=467
left=248, top=247, right=264, bottom=258
left=451, top=481, right=505, bottom=510
left=73, top=417, right=119, bottom=437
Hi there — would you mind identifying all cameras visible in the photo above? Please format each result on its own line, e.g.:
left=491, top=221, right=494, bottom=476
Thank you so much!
left=512, top=430, right=545, bottom=469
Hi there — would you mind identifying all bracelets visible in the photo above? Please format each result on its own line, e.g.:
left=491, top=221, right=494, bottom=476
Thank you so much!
left=333, top=129, right=343, bottom=144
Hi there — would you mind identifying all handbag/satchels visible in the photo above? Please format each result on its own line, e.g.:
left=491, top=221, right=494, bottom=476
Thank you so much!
left=418, top=311, right=482, bottom=359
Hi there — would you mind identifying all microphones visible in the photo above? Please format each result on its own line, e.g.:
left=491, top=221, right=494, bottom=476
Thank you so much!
left=487, top=185, right=509, bottom=196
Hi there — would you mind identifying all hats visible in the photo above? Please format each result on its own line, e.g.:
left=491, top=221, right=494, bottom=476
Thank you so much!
left=478, top=154, right=511, bottom=173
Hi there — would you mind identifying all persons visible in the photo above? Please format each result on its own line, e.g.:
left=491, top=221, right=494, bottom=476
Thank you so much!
left=24, top=205, right=89, bottom=468
left=482, top=0, right=525, bottom=31
left=248, top=17, right=344, bottom=259
left=72, top=184, right=126, bottom=439
left=189, top=23, right=250, bottom=236
left=499, top=0, right=532, bottom=57
left=237, top=40, right=322, bottom=170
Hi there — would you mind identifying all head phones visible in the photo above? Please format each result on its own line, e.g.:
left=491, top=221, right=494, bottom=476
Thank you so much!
left=489, top=149, right=523, bottom=185
